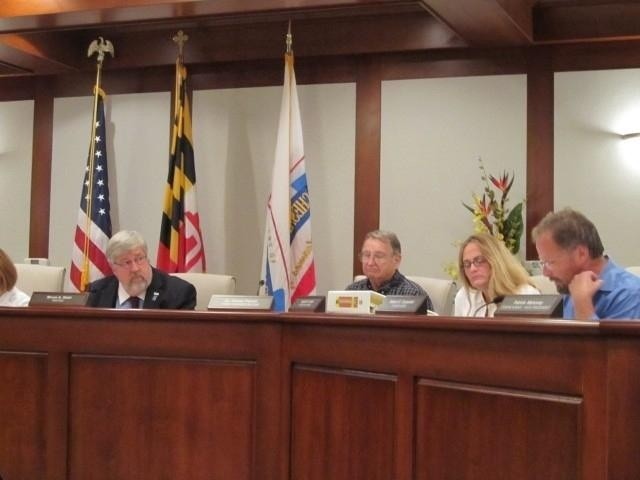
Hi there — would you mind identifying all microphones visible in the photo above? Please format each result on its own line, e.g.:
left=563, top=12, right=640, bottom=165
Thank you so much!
left=255, top=280, right=264, bottom=296
left=471, top=295, right=504, bottom=318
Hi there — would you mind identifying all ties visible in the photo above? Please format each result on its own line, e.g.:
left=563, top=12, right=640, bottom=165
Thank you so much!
left=130, top=297, right=139, bottom=308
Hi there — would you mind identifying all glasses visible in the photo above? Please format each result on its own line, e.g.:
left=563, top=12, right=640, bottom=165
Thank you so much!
left=114, top=254, right=147, bottom=268
left=460, top=258, right=486, bottom=267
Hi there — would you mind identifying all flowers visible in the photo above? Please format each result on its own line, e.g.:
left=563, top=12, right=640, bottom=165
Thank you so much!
left=464, top=157, right=517, bottom=248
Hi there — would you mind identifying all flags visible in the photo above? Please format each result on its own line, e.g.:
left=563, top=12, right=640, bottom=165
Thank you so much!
left=256, top=50, right=318, bottom=311
left=64, top=84, right=112, bottom=294
left=156, top=54, right=207, bottom=274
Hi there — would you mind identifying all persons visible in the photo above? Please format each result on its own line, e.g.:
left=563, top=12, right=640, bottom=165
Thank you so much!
left=531, top=208, right=640, bottom=321
left=84, top=230, right=197, bottom=310
left=454, top=233, right=541, bottom=317
left=0, top=248, right=30, bottom=307
left=344, top=231, right=434, bottom=313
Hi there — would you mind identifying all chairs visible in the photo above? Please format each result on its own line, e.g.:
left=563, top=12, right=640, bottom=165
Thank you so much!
left=169, top=271, right=237, bottom=311
left=626, top=266, right=640, bottom=277
left=532, top=275, right=560, bottom=295
left=12, top=257, right=65, bottom=299
left=354, top=275, right=454, bottom=316
